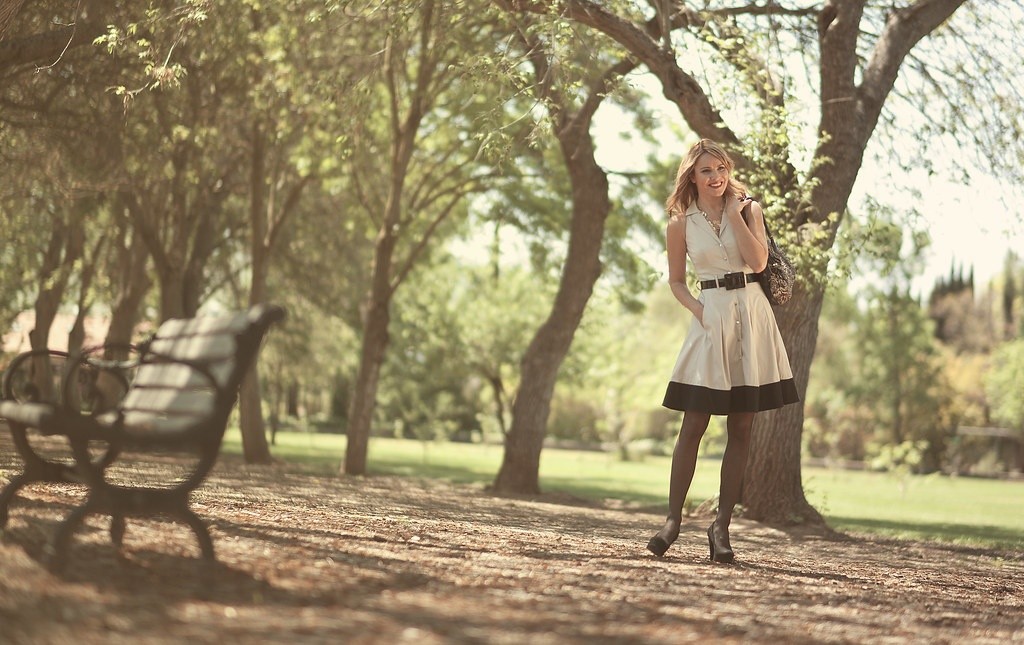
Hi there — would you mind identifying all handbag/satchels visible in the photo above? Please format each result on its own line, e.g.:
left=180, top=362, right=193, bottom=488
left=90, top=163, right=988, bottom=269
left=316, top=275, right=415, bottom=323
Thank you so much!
left=740, top=197, right=794, bottom=304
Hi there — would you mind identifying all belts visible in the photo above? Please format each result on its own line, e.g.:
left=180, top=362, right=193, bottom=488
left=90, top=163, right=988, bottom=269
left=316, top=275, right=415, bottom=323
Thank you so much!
left=700, top=273, right=760, bottom=290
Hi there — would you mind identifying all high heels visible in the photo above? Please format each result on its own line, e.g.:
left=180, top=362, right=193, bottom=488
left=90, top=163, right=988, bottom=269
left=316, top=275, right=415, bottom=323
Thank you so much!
left=647, top=531, right=679, bottom=556
left=707, top=521, right=734, bottom=563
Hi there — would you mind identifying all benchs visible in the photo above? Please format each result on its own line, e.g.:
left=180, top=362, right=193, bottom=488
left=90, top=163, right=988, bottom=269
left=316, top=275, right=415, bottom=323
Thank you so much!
left=0, top=301, right=288, bottom=596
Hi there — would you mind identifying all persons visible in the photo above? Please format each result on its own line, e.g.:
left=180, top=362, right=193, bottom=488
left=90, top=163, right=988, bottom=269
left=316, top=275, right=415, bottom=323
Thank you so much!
left=646, top=138, right=801, bottom=560
